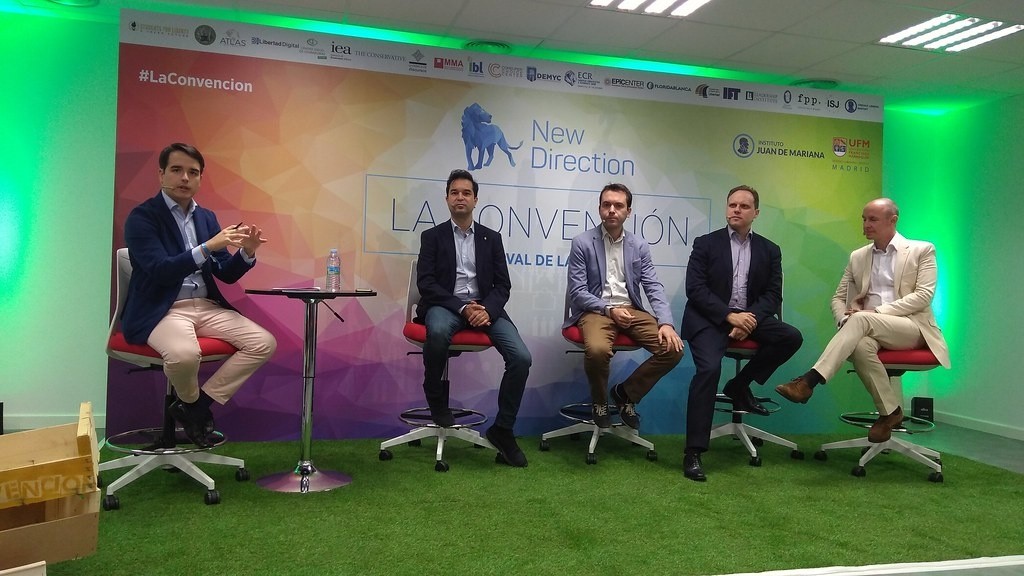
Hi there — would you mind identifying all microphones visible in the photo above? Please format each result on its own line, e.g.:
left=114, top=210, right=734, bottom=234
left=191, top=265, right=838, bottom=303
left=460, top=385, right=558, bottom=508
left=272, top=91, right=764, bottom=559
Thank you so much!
left=603, top=220, right=606, bottom=222
left=163, top=186, right=176, bottom=190
left=863, top=232, right=865, bottom=235
left=451, top=204, right=454, bottom=207
left=726, top=216, right=732, bottom=219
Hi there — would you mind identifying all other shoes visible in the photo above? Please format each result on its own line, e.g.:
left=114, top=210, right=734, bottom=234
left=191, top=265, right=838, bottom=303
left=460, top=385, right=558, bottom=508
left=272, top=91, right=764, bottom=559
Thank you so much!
left=486, top=424, right=528, bottom=467
left=431, top=408, right=456, bottom=427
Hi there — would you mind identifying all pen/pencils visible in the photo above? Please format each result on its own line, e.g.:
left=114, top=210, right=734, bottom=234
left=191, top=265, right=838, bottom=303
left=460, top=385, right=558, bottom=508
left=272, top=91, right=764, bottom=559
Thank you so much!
left=233, top=222, right=243, bottom=229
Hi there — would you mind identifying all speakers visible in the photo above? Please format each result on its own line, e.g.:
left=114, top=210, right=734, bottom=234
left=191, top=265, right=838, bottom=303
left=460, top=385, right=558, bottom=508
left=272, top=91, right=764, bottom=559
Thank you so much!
left=910, top=396, right=933, bottom=424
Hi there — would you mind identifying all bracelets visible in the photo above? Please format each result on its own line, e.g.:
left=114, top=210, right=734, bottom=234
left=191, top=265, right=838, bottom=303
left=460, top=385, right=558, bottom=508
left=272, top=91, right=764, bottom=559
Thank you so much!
left=202, top=242, right=210, bottom=255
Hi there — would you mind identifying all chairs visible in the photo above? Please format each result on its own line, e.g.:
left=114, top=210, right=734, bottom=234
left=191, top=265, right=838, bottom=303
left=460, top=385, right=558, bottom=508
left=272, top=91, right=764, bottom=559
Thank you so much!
left=700, top=270, right=804, bottom=465
left=380, top=259, right=509, bottom=474
left=538, top=271, right=656, bottom=463
left=90, top=247, right=250, bottom=509
left=813, top=346, right=944, bottom=482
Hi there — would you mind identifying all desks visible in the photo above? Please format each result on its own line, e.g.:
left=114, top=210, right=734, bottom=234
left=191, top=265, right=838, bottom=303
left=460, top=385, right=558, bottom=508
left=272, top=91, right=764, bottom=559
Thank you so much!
left=243, top=289, right=376, bottom=494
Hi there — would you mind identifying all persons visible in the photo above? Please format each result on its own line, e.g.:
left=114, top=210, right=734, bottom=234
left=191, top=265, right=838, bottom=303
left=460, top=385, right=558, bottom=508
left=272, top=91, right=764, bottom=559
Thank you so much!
left=775, top=198, right=952, bottom=443
left=119, top=143, right=277, bottom=449
left=562, top=184, right=684, bottom=430
left=679, top=185, right=802, bottom=481
left=416, top=170, right=532, bottom=466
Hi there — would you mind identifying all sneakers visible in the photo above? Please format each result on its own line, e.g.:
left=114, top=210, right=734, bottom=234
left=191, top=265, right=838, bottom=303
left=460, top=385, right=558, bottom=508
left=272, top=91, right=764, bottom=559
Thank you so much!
left=609, top=383, right=641, bottom=430
left=592, top=399, right=612, bottom=429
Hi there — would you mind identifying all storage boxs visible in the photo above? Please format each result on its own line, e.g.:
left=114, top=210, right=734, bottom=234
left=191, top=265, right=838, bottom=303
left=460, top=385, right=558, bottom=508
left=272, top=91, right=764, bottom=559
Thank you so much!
left=0, top=400, right=102, bottom=569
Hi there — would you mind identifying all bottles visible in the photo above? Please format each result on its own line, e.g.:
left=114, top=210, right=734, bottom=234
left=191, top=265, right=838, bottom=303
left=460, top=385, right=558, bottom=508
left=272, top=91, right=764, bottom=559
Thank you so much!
left=326, top=249, right=341, bottom=292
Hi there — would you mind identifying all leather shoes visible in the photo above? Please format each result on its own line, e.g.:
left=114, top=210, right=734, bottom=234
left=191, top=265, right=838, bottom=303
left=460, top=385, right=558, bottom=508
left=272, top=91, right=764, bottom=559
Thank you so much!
left=168, top=401, right=212, bottom=447
left=683, top=454, right=707, bottom=481
left=775, top=377, right=812, bottom=404
left=186, top=407, right=226, bottom=443
left=866, top=406, right=903, bottom=443
left=723, top=379, right=770, bottom=416
